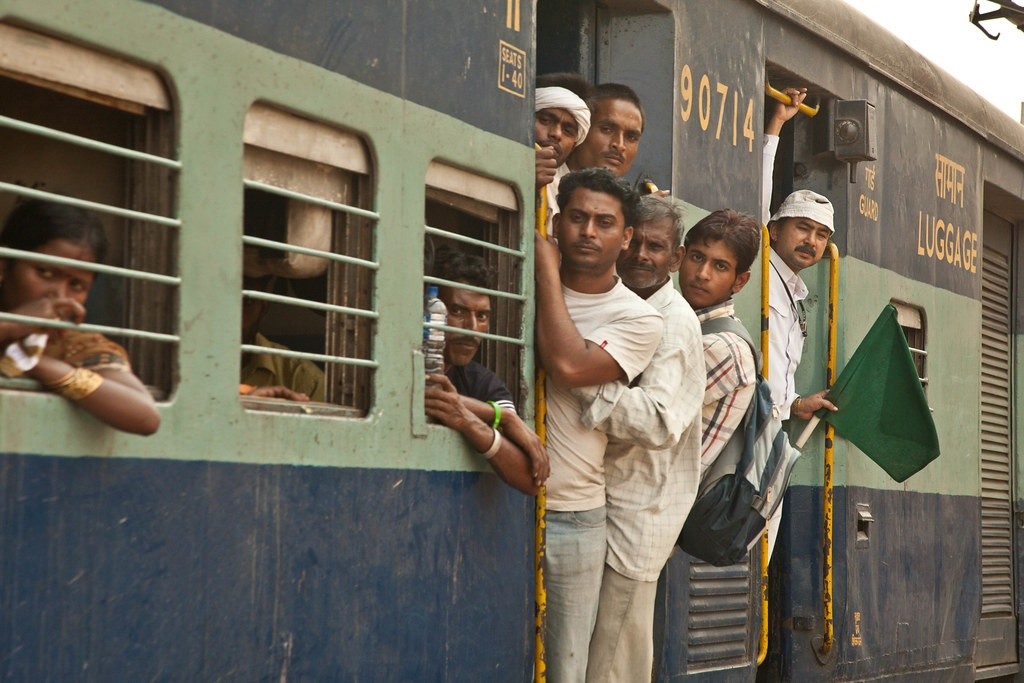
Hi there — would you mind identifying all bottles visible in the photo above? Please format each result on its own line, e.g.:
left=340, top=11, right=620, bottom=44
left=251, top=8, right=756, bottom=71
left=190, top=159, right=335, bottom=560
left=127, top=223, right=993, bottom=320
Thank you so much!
left=422, top=286, right=447, bottom=391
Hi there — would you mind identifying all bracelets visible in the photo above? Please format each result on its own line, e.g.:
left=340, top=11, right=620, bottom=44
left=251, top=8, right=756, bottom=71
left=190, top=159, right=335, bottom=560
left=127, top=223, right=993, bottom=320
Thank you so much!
left=485, top=399, right=501, bottom=430
left=474, top=430, right=501, bottom=459
left=48, top=366, right=103, bottom=401
left=796, top=398, right=803, bottom=417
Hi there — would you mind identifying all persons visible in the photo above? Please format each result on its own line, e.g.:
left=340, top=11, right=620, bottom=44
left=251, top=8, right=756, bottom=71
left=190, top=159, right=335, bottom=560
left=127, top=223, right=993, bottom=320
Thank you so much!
left=0, top=182, right=162, bottom=436
left=671, top=206, right=759, bottom=487
left=232, top=256, right=328, bottom=404
left=522, top=72, right=706, bottom=683
left=756, top=84, right=841, bottom=573
left=427, top=244, right=550, bottom=497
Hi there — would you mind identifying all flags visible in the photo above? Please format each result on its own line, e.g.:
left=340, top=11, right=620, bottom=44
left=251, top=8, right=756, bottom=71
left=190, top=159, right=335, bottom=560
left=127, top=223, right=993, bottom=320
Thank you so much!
left=816, top=301, right=942, bottom=484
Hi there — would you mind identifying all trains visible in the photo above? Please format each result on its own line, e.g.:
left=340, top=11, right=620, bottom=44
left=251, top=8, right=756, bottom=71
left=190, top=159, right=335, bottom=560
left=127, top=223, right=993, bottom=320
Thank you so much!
left=0, top=2, right=1024, bottom=683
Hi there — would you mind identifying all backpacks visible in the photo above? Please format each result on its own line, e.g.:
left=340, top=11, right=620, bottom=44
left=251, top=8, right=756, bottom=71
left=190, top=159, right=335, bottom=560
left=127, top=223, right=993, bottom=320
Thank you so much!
left=674, top=317, right=802, bottom=566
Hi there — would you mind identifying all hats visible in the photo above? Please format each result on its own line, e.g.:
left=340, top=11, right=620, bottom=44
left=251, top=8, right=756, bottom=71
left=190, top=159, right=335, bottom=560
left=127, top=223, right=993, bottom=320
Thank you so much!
left=766, top=190, right=835, bottom=238
